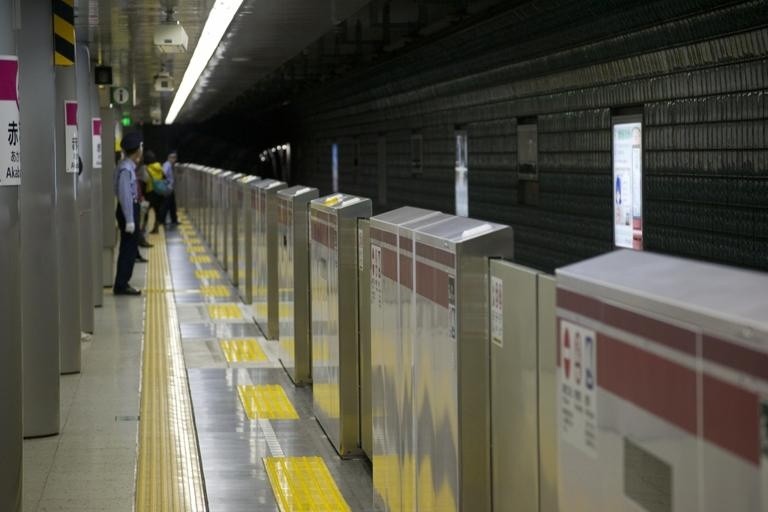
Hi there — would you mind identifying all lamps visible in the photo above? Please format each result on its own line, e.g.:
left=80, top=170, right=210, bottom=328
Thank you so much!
left=149, top=101, right=164, bottom=126
left=153, top=63, right=178, bottom=93
left=152, top=10, right=188, bottom=54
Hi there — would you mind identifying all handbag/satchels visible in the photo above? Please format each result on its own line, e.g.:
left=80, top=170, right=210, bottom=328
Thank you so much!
left=153, top=182, right=168, bottom=194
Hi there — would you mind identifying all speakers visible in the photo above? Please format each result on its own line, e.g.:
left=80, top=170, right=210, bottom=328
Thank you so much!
left=95, top=64, right=113, bottom=84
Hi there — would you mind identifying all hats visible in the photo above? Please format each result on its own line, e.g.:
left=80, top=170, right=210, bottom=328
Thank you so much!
left=120, top=131, right=143, bottom=148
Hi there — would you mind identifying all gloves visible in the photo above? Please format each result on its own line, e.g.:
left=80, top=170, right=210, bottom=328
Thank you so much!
left=126, top=222, right=135, bottom=232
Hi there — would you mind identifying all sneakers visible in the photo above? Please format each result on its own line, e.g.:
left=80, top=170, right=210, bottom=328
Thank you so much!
left=114, top=287, right=141, bottom=295
left=171, top=221, right=181, bottom=225
left=149, top=229, right=158, bottom=233
left=136, top=257, right=148, bottom=263
left=139, top=243, right=154, bottom=247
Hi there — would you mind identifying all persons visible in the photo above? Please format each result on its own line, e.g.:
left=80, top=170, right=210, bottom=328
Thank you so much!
left=113, top=132, right=144, bottom=294
left=119, top=150, right=183, bottom=262
